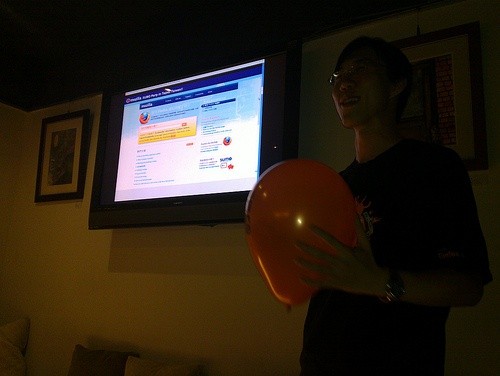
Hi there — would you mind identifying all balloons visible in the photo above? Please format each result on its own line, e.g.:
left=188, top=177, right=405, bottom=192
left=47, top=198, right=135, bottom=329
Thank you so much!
left=244, top=158, right=360, bottom=311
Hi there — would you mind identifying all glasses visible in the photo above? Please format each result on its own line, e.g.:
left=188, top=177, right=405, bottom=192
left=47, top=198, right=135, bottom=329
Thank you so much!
left=328, top=63, right=388, bottom=89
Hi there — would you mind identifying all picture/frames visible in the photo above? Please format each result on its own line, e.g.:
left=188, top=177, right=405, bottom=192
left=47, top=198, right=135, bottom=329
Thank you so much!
left=387, top=19, right=488, bottom=172
left=34, top=109, right=87, bottom=203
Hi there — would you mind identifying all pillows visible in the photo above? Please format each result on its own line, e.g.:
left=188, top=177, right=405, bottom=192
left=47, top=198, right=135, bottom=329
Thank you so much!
left=124, top=356, right=205, bottom=376
left=67, top=344, right=140, bottom=376
left=0, top=317, right=31, bottom=376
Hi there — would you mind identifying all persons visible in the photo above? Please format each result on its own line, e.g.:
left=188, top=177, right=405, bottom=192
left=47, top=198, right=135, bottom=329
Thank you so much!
left=293, top=35, right=494, bottom=376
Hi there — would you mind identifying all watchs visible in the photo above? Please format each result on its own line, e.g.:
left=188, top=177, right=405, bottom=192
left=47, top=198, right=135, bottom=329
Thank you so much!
left=377, top=265, right=407, bottom=304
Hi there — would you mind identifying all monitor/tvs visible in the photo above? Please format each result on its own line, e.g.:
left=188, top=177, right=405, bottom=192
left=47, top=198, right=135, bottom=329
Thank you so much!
left=88, top=40, right=302, bottom=230
